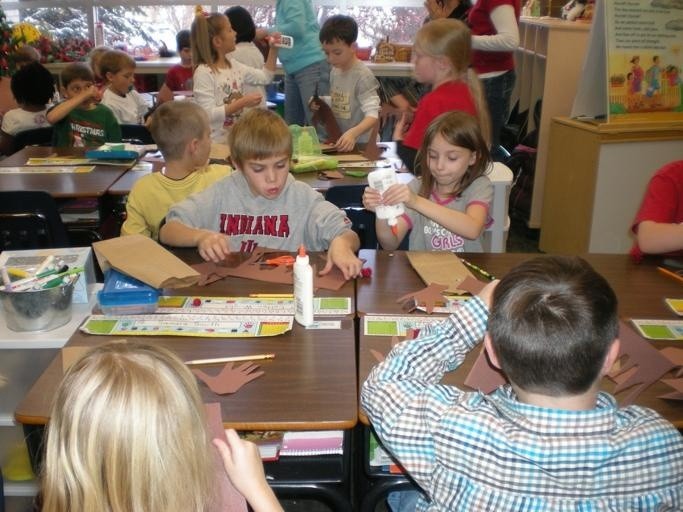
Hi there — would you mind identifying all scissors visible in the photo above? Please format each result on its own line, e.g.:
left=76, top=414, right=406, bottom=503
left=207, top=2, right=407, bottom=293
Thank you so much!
left=253, top=256, right=295, bottom=266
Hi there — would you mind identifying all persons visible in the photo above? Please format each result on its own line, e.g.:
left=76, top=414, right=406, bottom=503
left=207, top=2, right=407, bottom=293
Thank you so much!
left=360, top=257, right=682, bottom=512
left=392, top=17, right=493, bottom=178
left=0, top=63, right=56, bottom=157
left=0, top=45, right=41, bottom=117
left=98, top=50, right=173, bottom=127
left=119, top=100, right=234, bottom=243
left=468, top=0, right=521, bottom=146
left=41, top=342, right=285, bottom=512
left=630, top=160, right=683, bottom=264
left=158, top=108, right=364, bottom=281
left=89, top=46, right=114, bottom=104
left=165, top=29, right=196, bottom=92
left=422, top=0, right=474, bottom=25
left=224, top=5, right=267, bottom=110
left=253, top=0, right=331, bottom=143
left=46, top=63, right=122, bottom=148
left=308, top=14, right=381, bottom=153
left=362, top=111, right=495, bottom=254
left=190, top=5, right=281, bottom=144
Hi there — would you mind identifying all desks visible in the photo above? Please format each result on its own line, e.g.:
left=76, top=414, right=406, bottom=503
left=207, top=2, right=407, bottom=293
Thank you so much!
left=355, top=249, right=683, bottom=314
left=14, top=315, right=358, bottom=512
left=40, top=51, right=415, bottom=97
left=137, top=89, right=285, bottom=119
left=0, top=143, right=140, bottom=247
left=107, top=144, right=411, bottom=237
left=91, top=246, right=355, bottom=315
left=358, top=314, right=683, bottom=512
left=537, top=111, right=683, bottom=255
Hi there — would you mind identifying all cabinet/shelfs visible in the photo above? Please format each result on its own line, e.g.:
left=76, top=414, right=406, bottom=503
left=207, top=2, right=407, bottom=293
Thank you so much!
left=507, top=16, right=593, bottom=230
left=0, top=281, right=104, bottom=512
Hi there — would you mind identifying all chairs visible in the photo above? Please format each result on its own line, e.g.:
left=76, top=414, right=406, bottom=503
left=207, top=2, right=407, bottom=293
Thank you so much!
left=325, top=184, right=377, bottom=251
left=0, top=191, right=72, bottom=253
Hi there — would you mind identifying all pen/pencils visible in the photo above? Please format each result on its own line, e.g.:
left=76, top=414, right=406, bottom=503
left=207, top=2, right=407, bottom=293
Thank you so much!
left=250, top=294, right=315, bottom=298
left=184, top=353, right=276, bottom=365
left=657, top=267, right=683, bottom=282
left=459, top=258, right=496, bottom=281
left=0, top=260, right=82, bottom=292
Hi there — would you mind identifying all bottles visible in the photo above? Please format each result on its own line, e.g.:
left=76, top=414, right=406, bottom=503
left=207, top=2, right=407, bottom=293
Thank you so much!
left=293, top=244, right=314, bottom=327
left=368, top=168, right=404, bottom=235
left=264, top=34, right=294, bottom=48
